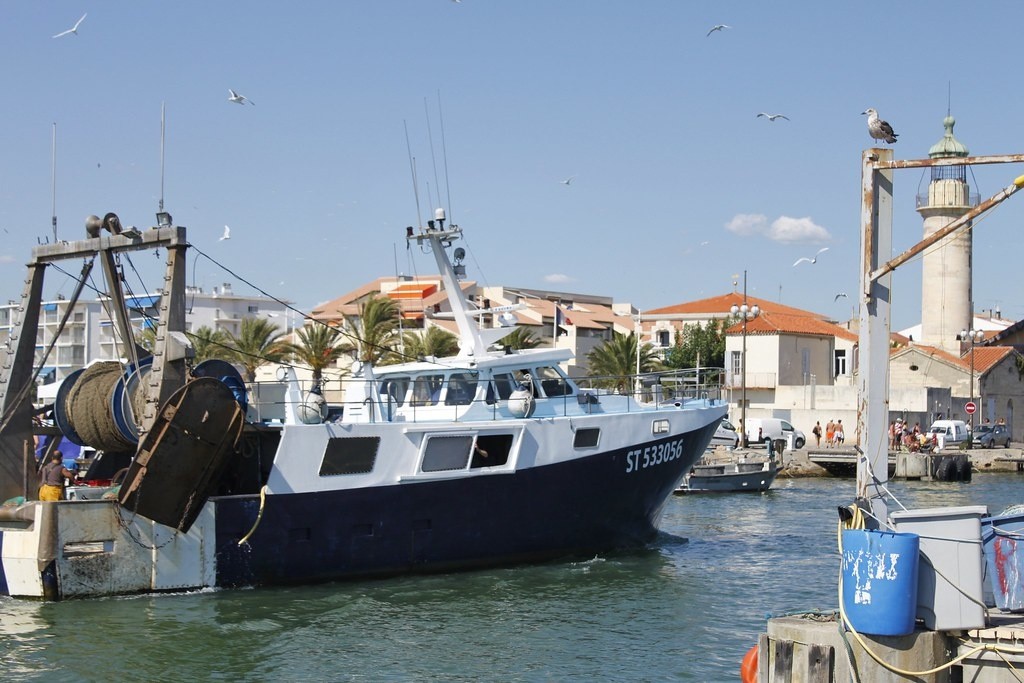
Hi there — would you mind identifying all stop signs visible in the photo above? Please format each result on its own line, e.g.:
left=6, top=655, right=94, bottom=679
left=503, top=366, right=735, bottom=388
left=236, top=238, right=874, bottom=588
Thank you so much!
left=965, top=402, right=976, bottom=414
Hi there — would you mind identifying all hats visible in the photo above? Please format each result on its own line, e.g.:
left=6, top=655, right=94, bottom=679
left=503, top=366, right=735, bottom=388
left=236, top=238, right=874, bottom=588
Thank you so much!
left=897, top=418, right=903, bottom=422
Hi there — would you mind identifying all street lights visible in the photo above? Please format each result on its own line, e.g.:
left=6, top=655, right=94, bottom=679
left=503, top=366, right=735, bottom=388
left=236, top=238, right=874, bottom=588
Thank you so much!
left=960, top=328, right=983, bottom=447
left=728, top=302, right=759, bottom=447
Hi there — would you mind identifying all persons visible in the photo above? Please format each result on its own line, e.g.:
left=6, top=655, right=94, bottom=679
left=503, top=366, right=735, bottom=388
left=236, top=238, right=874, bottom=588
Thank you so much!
left=38, top=450, right=82, bottom=500
left=737, top=419, right=743, bottom=447
left=814, top=418, right=1006, bottom=450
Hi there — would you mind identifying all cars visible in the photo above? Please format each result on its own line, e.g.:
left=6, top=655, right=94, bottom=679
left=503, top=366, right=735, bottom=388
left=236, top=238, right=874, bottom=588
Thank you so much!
left=968, top=425, right=1011, bottom=449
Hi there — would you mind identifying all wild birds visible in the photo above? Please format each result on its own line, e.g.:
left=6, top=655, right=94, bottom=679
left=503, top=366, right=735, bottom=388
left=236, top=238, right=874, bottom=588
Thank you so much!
left=861, top=109, right=899, bottom=147
left=228, top=89, right=255, bottom=105
left=755, top=113, right=790, bottom=122
left=561, top=177, right=574, bottom=185
left=706, top=24, right=732, bottom=36
left=834, top=294, right=847, bottom=302
left=217, top=226, right=231, bottom=243
left=51, top=13, right=88, bottom=39
left=792, top=248, right=829, bottom=267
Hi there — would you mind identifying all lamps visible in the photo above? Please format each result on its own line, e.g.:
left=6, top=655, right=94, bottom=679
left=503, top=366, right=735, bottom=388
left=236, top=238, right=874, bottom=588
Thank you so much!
left=156, top=212, right=172, bottom=228
left=120, top=226, right=142, bottom=239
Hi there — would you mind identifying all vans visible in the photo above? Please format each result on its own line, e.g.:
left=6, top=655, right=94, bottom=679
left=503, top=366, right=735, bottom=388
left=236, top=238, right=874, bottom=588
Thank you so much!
left=710, top=419, right=739, bottom=449
left=926, top=420, right=967, bottom=447
left=746, top=419, right=805, bottom=449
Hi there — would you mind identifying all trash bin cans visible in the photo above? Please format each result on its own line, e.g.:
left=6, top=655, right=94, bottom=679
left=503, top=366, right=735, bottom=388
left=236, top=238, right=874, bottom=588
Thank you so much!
left=890, top=506, right=989, bottom=630
left=982, top=514, right=1024, bottom=611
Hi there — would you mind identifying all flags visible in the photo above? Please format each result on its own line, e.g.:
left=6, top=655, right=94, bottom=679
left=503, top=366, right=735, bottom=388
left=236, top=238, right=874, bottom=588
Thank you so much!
left=556, top=306, right=573, bottom=325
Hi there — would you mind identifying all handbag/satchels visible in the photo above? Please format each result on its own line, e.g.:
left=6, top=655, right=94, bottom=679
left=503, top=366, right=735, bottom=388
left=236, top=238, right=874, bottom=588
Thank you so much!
left=813, top=427, right=818, bottom=434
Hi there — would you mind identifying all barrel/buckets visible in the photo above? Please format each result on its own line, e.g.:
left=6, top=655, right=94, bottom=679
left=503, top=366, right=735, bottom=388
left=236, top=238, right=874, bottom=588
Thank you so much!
left=841, top=505, right=1024, bottom=637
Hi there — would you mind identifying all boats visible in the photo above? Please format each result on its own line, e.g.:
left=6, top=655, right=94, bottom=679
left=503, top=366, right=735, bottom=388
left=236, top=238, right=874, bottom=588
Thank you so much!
left=0, top=87, right=728, bottom=599
left=676, top=459, right=784, bottom=493
